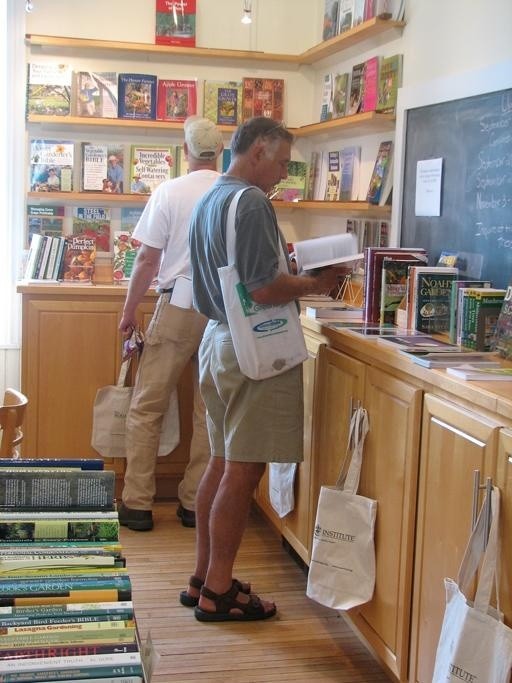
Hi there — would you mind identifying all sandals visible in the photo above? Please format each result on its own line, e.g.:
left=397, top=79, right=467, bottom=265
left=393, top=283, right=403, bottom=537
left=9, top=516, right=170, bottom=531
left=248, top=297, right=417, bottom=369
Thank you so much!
left=195, top=584, right=278, bottom=623
left=180, top=575, right=252, bottom=609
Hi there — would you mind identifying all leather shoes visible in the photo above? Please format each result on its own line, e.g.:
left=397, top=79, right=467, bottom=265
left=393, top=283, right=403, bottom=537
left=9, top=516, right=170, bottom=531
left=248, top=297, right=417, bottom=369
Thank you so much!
left=118, top=501, right=155, bottom=532
left=177, top=503, right=197, bottom=528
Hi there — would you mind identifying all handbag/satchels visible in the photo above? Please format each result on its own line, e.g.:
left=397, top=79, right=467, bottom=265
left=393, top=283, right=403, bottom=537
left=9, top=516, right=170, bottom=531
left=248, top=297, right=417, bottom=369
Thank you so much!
left=268, top=462, right=298, bottom=520
left=91, top=333, right=181, bottom=458
left=306, top=407, right=379, bottom=613
left=430, top=485, right=512, bottom=683
left=217, top=185, right=310, bottom=380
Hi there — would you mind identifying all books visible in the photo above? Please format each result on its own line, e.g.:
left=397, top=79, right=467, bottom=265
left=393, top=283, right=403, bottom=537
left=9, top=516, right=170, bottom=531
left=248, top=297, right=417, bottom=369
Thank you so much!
left=29, top=140, right=231, bottom=193
left=301, top=244, right=512, bottom=383
left=0, top=456, right=147, bottom=682
left=323, top=1, right=405, bottom=38
left=288, top=233, right=365, bottom=277
left=21, top=206, right=159, bottom=286
left=320, top=54, right=404, bottom=120
left=267, top=138, right=393, bottom=208
left=25, top=62, right=284, bottom=126
left=155, top=1, right=196, bottom=47
left=347, top=219, right=390, bottom=257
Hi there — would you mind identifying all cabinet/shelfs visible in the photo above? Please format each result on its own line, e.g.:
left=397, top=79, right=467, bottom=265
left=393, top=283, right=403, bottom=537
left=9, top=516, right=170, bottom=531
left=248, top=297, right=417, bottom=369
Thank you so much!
left=254, top=308, right=309, bottom=569
left=25, top=15, right=406, bottom=290
left=18, top=284, right=205, bottom=501
left=311, top=329, right=412, bottom=678
left=407, top=374, right=511, bottom=683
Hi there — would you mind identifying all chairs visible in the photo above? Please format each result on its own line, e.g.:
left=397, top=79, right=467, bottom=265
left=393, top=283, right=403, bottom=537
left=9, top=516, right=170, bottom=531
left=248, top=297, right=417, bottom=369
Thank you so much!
left=0, top=387, right=28, bottom=460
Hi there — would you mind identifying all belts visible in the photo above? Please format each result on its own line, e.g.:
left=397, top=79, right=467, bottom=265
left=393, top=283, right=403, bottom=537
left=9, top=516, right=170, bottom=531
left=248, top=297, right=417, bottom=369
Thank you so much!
left=161, top=289, right=174, bottom=294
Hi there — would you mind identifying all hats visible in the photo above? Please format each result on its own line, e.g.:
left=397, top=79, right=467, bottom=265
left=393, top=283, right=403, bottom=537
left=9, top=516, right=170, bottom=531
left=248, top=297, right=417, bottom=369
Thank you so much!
left=132, top=173, right=141, bottom=179
left=184, top=115, right=224, bottom=161
left=108, top=155, right=119, bottom=163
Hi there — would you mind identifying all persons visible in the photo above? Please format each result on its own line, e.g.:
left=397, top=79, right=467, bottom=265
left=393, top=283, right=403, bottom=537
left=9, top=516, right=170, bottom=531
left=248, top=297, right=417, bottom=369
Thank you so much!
left=117, top=117, right=225, bottom=532
left=180, top=115, right=353, bottom=621
